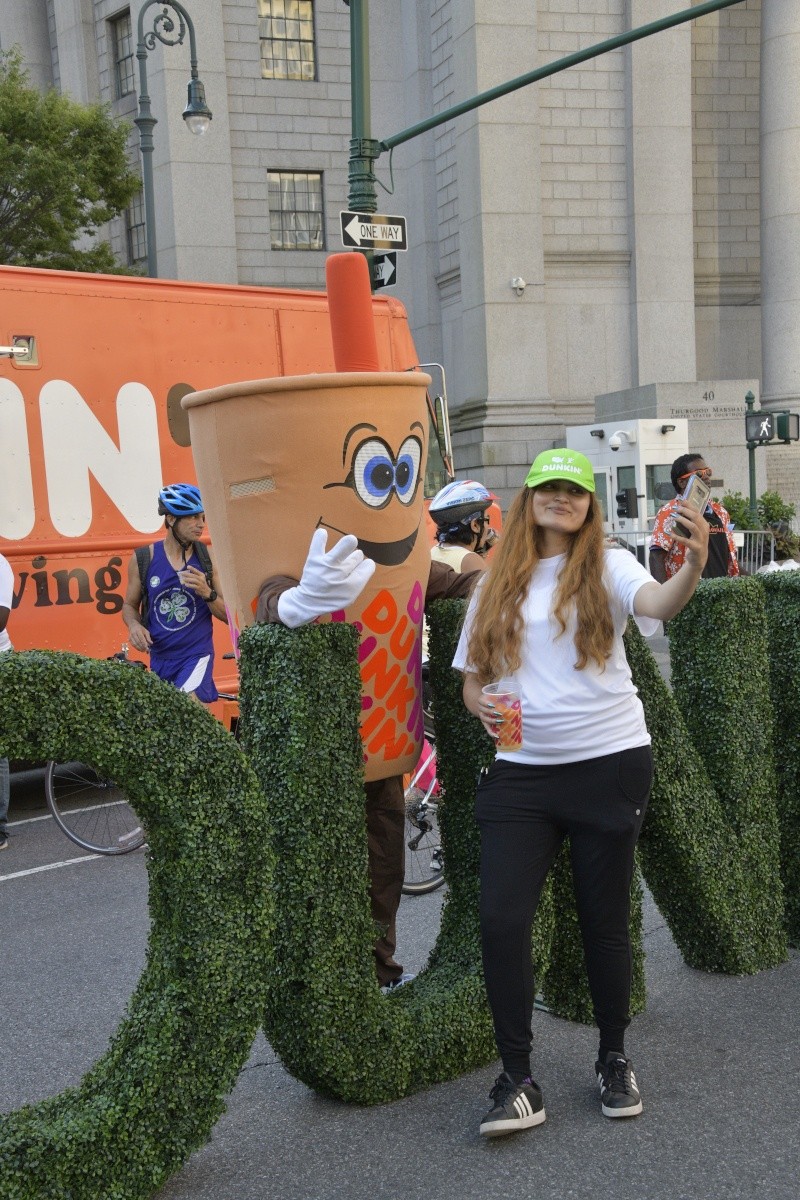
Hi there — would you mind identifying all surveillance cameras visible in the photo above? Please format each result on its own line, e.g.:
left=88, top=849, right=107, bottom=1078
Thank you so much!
left=517, top=281, right=525, bottom=290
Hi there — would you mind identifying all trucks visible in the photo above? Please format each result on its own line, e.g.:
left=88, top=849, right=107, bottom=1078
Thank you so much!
left=0, top=265, right=502, bottom=786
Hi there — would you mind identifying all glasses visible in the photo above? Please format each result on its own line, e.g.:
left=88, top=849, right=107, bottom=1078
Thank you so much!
left=680, top=468, right=712, bottom=480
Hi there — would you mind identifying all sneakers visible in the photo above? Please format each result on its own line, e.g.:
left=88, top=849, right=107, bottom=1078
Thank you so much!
left=479, top=1072, right=546, bottom=1137
left=595, top=1052, right=642, bottom=1117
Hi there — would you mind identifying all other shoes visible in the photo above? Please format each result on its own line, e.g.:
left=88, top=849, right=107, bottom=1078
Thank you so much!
left=380, top=974, right=416, bottom=995
left=0, top=832, right=10, bottom=849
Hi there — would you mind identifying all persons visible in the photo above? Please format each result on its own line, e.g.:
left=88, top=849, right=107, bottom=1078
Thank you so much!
left=648, top=455, right=741, bottom=584
left=0, top=554, right=14, bottom=850
left=449, top=447, right=711, bottom=1137
left=121, top=483, right=229, bottom=704
left=421, top=479, right=500, bottom=720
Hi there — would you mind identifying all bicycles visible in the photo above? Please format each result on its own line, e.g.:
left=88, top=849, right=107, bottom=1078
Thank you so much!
left=399, top=661, right=450, bottom=897
left=43, top=654, right=241, bottom=857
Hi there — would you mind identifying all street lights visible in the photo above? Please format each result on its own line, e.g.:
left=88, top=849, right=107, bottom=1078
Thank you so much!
left=133, top=1, right=214, bottom=279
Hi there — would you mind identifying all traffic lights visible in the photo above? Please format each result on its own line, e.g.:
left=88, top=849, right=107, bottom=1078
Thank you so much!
left=777, top=412, right=800, bottom=441
left=616, top=487, right=638, bottom=519
left=744, top=413, right=775, bottom=442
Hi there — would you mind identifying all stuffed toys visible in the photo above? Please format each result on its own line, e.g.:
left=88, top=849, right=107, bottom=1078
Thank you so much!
left=180, top=253, right=485, bottom=995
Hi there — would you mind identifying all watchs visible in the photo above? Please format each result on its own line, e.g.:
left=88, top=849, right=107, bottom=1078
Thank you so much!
left=202, top=588, right=217, bottom=602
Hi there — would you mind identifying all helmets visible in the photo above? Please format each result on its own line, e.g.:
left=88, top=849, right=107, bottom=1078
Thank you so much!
left=428, top=479, right=494, bottom=526
left=158, top=483, right=204, bottom=516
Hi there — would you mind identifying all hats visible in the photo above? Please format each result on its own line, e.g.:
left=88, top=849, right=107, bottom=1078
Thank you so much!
left=523, top=448, right=595, bottom=493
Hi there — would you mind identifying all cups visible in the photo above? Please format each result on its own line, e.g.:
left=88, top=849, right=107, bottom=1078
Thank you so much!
left=481, top=681, right=524, bottom=753
left=178, top=369, right=435, bottom=783
left=177, top=570, right=190, bottom=584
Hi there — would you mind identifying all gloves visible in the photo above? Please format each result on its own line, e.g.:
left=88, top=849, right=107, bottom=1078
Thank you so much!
left=278, top=528, right=377, bottom=630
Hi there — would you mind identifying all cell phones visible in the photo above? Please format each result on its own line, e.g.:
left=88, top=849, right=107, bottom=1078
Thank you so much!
left=671, top=475, right=711, bottom=538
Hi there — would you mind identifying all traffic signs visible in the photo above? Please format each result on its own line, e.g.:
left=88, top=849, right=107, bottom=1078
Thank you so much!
left=339, top=211, right=408, bottom=252
left=371, top=251, right=397, bottom=291
